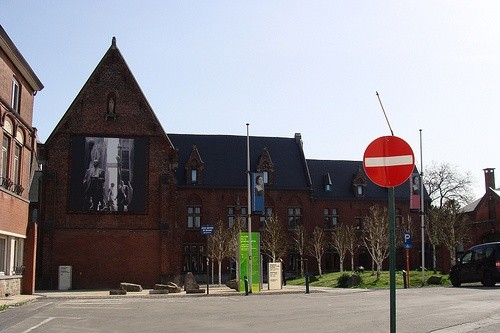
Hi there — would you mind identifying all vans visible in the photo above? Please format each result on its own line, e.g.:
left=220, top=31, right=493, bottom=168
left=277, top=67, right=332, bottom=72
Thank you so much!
left=450, top=242, right=500, bottom=287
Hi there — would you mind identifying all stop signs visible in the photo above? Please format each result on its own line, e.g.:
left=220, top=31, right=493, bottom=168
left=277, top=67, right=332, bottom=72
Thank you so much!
left=363, top=137, right=414, bottom=188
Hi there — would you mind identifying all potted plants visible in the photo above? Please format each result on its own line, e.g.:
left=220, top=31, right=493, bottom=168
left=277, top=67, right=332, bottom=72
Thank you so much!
left=13, top=266, right=26, bottom=275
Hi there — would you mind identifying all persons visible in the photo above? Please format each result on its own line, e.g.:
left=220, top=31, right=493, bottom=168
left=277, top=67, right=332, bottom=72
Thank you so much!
left=412, top=176, right=419, bottom=194
left=85, top=140, right=135, bottom=211
left=255, top=175, right=263, bottom=195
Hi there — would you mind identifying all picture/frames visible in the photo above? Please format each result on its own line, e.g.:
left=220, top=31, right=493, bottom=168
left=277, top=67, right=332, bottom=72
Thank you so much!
left=67, top=132, right=149, bottom=216
